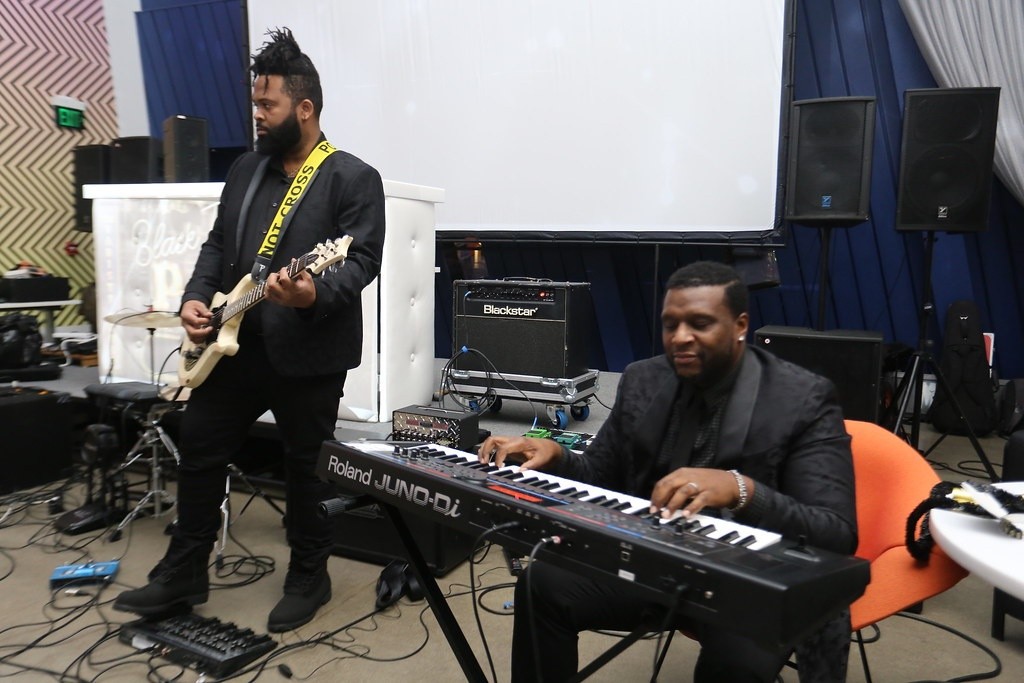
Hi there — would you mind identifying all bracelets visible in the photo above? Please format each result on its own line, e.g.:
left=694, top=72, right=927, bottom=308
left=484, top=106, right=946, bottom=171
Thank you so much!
left=728, top=470, right=747, bottom=512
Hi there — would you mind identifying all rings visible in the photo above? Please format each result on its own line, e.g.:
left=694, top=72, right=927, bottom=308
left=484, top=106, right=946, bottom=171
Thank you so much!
left=689, top=483, right=696, bottom=487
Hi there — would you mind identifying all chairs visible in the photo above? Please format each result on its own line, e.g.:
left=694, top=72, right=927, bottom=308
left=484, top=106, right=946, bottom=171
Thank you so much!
left=649, top=416, right=970, bottom=683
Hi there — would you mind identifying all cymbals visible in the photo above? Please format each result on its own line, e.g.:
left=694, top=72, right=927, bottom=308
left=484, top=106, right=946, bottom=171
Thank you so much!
left=102, top=310, right=185, bottom=330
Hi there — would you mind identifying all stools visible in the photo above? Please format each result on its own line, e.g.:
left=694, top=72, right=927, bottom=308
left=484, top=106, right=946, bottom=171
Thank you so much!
left=83, top=381, right=183, bottom=543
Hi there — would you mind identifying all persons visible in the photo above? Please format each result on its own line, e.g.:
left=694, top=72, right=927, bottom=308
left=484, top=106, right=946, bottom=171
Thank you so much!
left=112, top=29, right=386, bottom=634
left=479, top=263, right=857, bottom=683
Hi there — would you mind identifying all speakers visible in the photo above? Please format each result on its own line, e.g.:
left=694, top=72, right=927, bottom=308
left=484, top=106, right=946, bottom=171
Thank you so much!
left=452, top=279, right=592, bottom=378
left=73, top=115, right=210, bottom=233
left=786, top=95, right=877, bottom=230
left=895, top=86, right=1001, bottom=234
left=756, top=324, right=884, bottom=424
left=328, top=483, right=487, bottom=578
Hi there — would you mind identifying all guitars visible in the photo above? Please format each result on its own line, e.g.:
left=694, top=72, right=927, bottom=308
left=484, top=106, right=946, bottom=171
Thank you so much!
left=176, top=232, right=355, bottom=389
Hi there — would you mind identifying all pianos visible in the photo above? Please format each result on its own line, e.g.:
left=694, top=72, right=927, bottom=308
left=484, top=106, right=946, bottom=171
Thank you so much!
left=315, top=433, right=873, bottom=683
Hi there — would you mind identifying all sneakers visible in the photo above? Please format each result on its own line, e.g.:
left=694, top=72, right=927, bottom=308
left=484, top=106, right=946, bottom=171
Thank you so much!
left=112, top=572, right=208, bottom=612
left=269, top=580, right=334, bottom=631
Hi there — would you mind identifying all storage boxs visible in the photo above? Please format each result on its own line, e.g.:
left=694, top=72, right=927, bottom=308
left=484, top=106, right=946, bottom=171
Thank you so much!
left=441, top=369, right=599, bottom=403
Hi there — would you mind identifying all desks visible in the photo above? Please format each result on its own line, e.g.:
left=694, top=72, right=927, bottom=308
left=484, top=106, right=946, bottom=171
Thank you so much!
left=930, top=481, right=1024, bottom=602
left=81, top=182, right=446, bottom=423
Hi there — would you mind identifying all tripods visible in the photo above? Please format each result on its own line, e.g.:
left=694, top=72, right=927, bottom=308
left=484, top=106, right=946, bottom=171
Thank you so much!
left=110, top=326, right=288, bottom=568
left=881, top=231, right=1003, bottom=483
